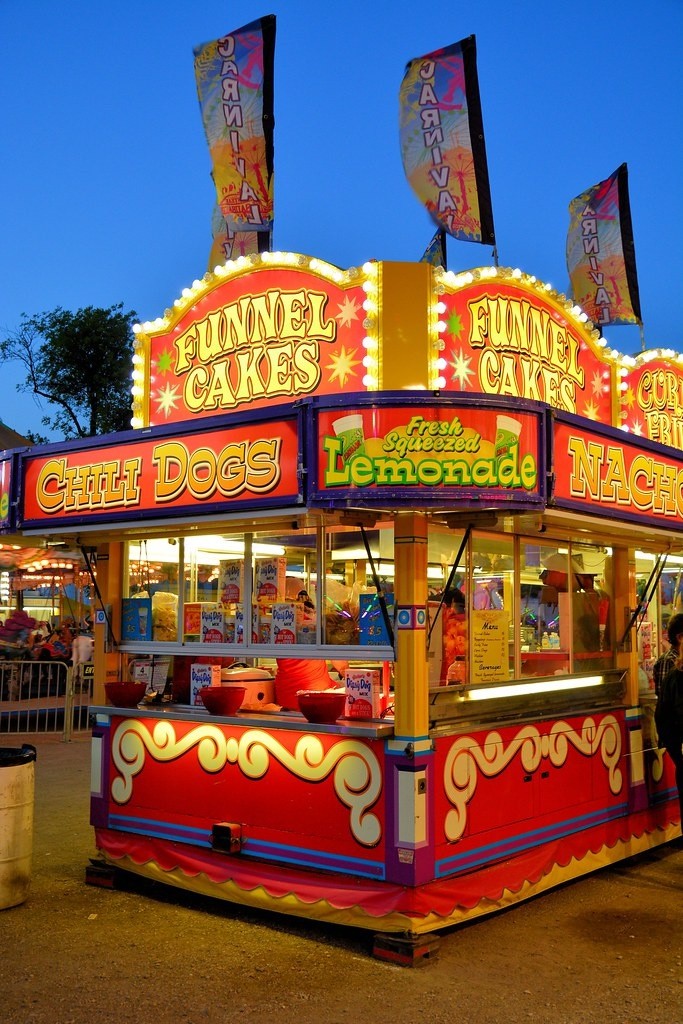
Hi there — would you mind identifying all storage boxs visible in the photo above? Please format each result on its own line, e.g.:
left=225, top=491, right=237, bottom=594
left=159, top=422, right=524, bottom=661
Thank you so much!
left=184, top=558, right=305, bottom=645
left=345, top=669, right=381, bottom=719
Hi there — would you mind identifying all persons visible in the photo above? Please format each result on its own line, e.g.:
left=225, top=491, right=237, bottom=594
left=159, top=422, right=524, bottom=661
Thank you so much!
left=86, top=620, right=94, bottom=634
left=436, top=587, right=466, bottom=614
left=654, top=613, right=683, bottom=834
left=298, top=589, right=313, bottom=608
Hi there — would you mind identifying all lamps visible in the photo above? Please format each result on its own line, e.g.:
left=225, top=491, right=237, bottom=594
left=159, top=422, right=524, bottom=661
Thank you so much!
left=532, top=516, right=683, bottom=555
left=74, top=514, right=303, bottom=545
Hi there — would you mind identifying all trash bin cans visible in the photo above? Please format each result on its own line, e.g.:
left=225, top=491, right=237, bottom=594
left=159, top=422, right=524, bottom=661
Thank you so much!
left=0, top=742, right=37, bottom=910
